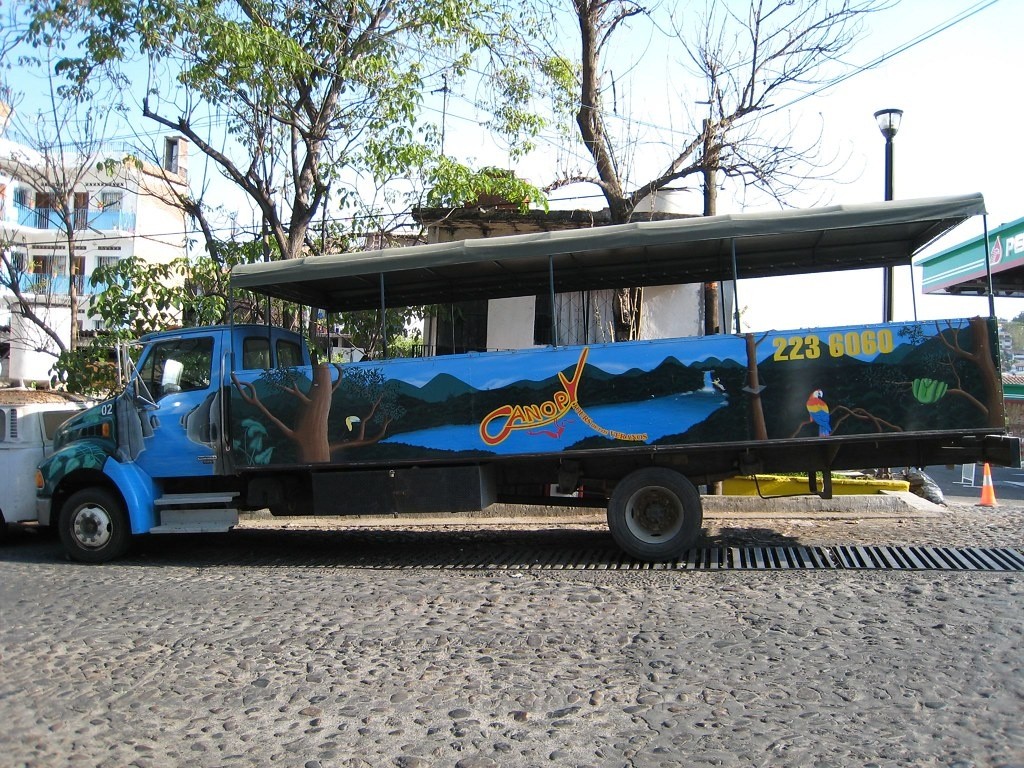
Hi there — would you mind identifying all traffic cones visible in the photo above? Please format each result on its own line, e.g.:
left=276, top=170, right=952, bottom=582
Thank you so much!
left=974, top=463, right=1002, bottom=508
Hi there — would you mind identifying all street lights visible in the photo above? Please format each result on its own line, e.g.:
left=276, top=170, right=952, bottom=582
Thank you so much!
left=872, top=104, right=906, bottom=322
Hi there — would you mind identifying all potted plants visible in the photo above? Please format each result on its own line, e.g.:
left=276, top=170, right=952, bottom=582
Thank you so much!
left=26, top=261, right=43, bottom=273
left=96, top=202, right=106, bottom=210
left=54, top=202, right=63, bottom=211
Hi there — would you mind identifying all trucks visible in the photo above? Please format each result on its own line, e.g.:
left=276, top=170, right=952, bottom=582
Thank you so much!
left=42, top=193, right=1005, bottom=556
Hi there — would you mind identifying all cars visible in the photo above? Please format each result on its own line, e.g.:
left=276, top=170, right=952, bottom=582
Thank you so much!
left=1, top=400, right=101, bottom=548
